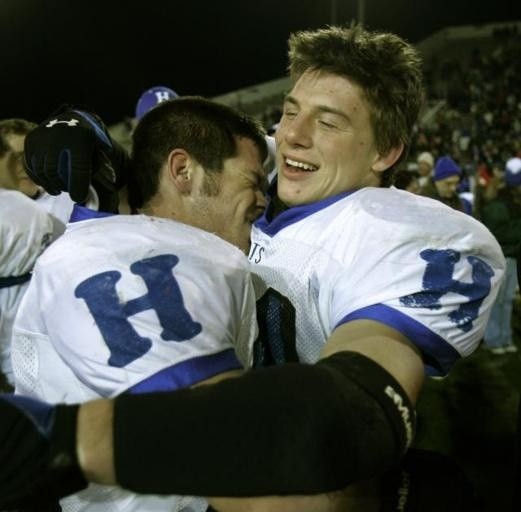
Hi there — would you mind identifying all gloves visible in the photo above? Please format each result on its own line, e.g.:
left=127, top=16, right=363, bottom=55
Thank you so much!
left=24, top=103, right=119, bottom=213
left=1, top=393, right=91, bottom=512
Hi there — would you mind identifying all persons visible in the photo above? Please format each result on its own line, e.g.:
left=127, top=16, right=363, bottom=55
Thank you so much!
left=1, top=189, right=68, bottom=396
left=395, top=22, right=520, bottom=260
left=2, top=25, right=507, bottom=510
left=12, top=96, right=351, bottom=510
left=0, top=119, right=76, bottom=225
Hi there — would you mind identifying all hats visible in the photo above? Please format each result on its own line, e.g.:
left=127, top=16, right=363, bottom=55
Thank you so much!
left=136, top=85, right=179, bottom=120
left=418, top=152, right=434, bottom=168
left=432, top=156, right=459, bottom=182
